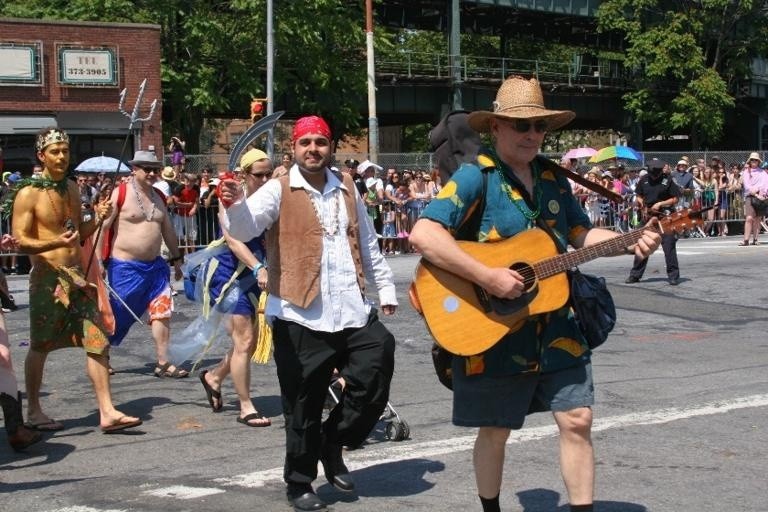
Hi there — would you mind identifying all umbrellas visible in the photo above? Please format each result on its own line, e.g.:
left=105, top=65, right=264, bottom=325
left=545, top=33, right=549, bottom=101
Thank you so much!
left=587, top=145, right=643, bottom=164
left=559, top=147, right=598, bottom=159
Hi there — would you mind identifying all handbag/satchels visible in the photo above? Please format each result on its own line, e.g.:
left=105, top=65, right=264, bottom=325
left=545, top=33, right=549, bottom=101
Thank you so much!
left=567, top=268, right=615, bottom=348
left=750, top=196, right=767, bottom=215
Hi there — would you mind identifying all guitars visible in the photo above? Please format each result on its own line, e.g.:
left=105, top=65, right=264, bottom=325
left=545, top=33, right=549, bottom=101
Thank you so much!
left=413, top=207, right=703, bottom=356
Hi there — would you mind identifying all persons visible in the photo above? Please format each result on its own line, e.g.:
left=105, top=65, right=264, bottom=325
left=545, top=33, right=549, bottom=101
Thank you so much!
left=196, top=148, right=278, bottom=428
left=215, top=114, right=402, bottom=511
left=345, top=159, right=443, bottom=254
left=0, top=166, right=44, bottom=274
left=405, top=76, right=664, bottom=512
left=168, top=135, right=187, bottom=174
left=153, top=154, right=293, bottom=256
left=10, top=128, right=144, bottom=435
left=625, top=160, right=680, bottom=284
left=95, top=149, right=192, bottom=380
left=77, top=173, right=133, bottom=221
left=0, top=271, right=45, bottom=455
left=560, top=152, right=768, bottom=246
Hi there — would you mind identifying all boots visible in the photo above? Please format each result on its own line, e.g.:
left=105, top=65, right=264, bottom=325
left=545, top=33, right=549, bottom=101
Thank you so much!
left=0, top=391, right=42, bottom=451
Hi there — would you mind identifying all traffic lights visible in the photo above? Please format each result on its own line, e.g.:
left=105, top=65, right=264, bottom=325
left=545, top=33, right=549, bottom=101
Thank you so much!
left=251, top=101, right=263, bottom=125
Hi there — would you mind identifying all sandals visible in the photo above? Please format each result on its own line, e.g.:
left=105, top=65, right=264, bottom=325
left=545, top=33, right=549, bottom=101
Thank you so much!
left=154, top=362, right=188, bottom=378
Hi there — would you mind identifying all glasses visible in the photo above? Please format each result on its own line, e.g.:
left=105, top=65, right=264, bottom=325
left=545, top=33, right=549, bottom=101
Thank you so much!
left=493, top=118, right=546, bottom=132
left=135, top=164, right=160, bottom=173
left=246, top=170, right=271, bottom=177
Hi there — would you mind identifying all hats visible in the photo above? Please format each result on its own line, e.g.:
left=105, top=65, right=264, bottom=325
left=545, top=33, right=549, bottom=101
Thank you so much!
left=345, top=159, right=358, bottom=165
left=587, top=164, right=625, bottom=180
left=645, top=160, right=663, bottom=176
left=467, top=75, right=576, bottom=132
left=676, top=160, right=688, bottom=167
left=127, top=151, right=162, bottom=166
left=745, top=152, right=761, bottom=163
left=161, top=166, right=176, bottom=180
left=365, top=177, right=377, bottom=189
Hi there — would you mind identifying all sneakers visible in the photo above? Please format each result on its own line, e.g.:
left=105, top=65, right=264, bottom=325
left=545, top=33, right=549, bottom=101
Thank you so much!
left=668, top=278, right=677, bottom=284
left=626, top=276, right=638, bottom=282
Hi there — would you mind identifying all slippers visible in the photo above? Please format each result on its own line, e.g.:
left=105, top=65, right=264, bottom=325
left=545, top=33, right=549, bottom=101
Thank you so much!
left=237, top=413, right=270, bottom=427
left=102, top=416, right=141, bottom=431
left=23, top=419, right=64, bottom=430
left=198, top=370, right=222, bottom=410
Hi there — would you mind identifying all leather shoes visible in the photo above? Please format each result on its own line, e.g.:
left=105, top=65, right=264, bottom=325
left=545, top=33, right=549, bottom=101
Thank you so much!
left=286, top=483, right=328, bottom=512
left=319, top=429, right=355, bottom=493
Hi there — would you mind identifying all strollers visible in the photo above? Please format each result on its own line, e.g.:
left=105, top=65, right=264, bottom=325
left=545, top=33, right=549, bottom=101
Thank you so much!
left=327, top=367, right=411, bottom=450
